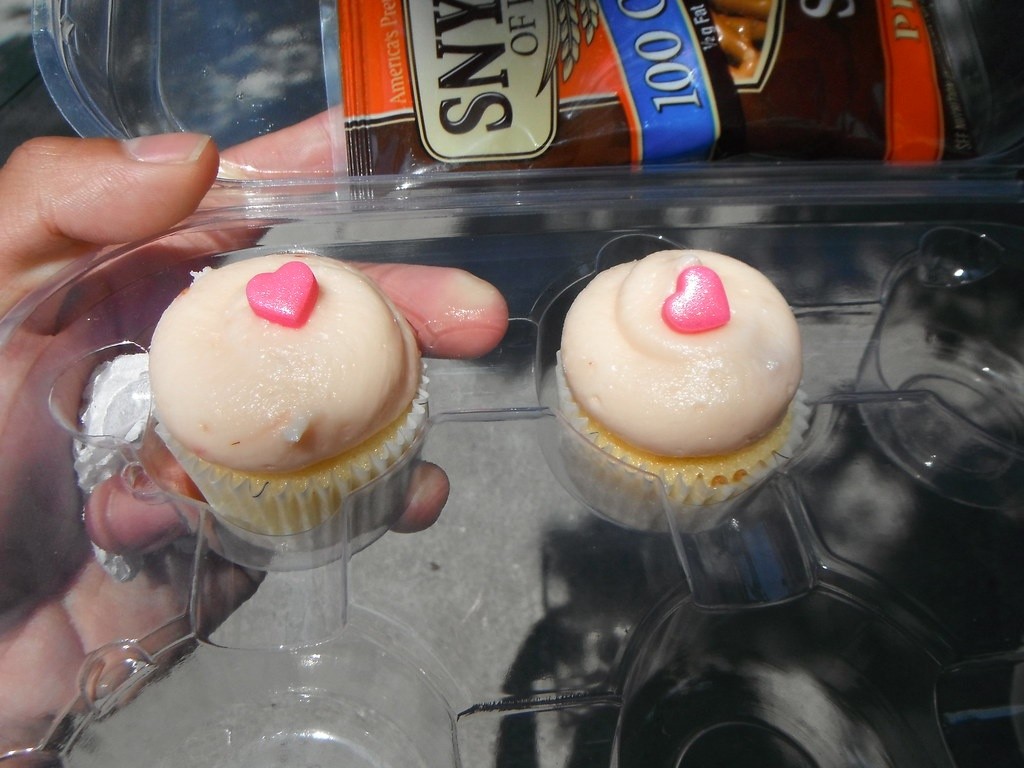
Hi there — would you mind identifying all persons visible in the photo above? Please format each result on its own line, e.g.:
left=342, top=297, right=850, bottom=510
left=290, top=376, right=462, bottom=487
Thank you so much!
left=1, top=133, right=511, bottom=767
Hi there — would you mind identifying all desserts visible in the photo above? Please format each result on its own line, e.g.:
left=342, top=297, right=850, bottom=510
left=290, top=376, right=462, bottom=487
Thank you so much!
left=555, top=247, right=812, bottom=528
left=146, top=252, right=431, bottom=549
